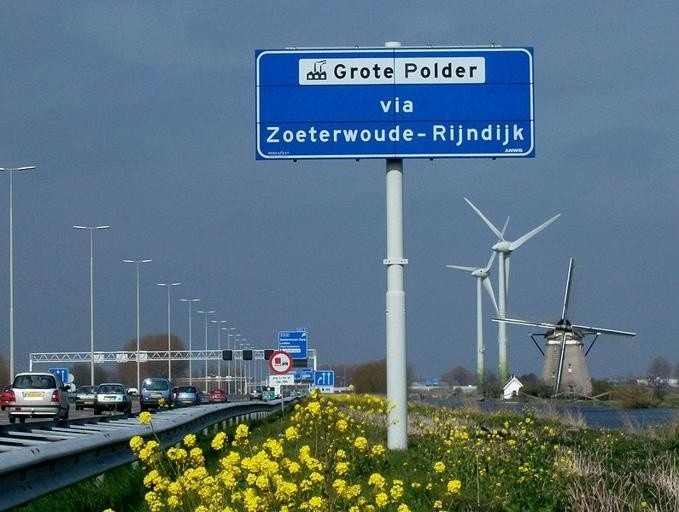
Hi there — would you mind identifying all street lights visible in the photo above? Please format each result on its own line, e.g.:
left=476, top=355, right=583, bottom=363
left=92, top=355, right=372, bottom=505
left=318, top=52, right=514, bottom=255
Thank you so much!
left=210, top=320, right=227, bottom=390
left=222, top=327, right=236, bottom=394
left=156, top=281, right=181, bottom=382
left=229, top=334, right=257, bottom=396
left=72, top=224, right=112, bottom=384
left=179, top=297, right=201, bottom=386
left=124, top=259, right=156, bottom=394
left=1, top=165, right=38, bottom=386
left=197, top=310, right=216, bottom=395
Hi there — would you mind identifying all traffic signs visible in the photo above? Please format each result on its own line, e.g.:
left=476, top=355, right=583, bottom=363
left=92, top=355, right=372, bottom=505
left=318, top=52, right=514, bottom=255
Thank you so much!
left=252, top=48, right=534, bottom=158
left=278, top=331, right=308, bottom=359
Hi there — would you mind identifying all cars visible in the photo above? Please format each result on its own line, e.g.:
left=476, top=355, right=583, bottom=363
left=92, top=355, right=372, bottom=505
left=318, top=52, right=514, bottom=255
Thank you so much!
left=293, top=367, right=314, bottom=383
left=250, top=388, right=304, bottom=401
left=1, top=372, right=231, bottom=421
